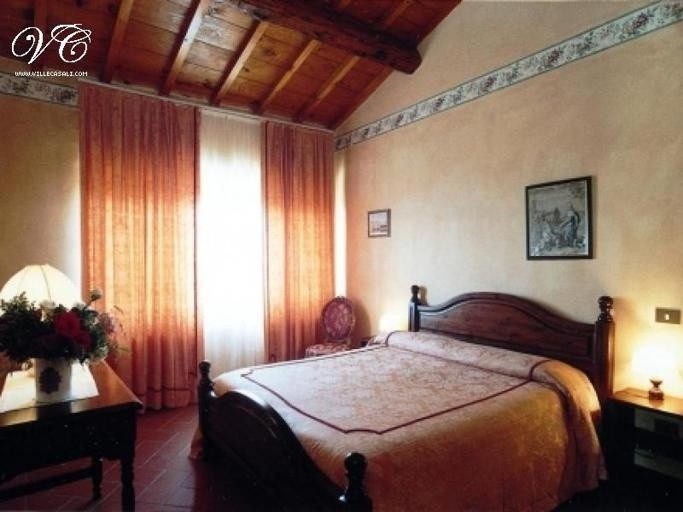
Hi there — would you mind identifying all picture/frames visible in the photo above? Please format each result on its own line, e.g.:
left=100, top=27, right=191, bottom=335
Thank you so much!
left=523, top=174, right=595, bottom=262
left=366, top=206, right=391, bottom=238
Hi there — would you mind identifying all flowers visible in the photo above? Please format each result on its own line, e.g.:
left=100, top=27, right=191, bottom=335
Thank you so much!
left=0, top=283, right=132, bottom=372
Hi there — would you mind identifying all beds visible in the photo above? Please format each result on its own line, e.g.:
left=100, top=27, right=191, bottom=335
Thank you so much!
left=186, top=283, right=617, bottom=511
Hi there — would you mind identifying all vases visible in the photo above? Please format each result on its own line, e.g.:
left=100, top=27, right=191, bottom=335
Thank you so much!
left=32, top=355, right=72, bottom=402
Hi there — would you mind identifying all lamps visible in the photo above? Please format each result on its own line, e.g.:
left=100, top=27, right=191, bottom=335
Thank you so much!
left=632, top=334, right=677, bottom=400
left=0, top=263, right=84, bottom=314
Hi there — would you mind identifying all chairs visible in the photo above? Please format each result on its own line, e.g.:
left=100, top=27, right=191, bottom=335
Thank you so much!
left=300, top=296, right=357, bottom=358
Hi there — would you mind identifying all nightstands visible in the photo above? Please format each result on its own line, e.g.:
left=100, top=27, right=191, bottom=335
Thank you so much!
left=604, top=386, right=682, bottom=511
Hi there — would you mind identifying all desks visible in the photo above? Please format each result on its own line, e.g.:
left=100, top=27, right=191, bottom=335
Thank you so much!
left=0, top=359, right=144, bottom=511
